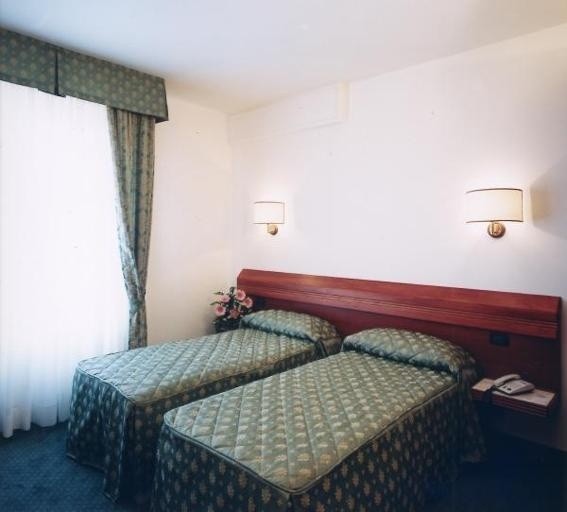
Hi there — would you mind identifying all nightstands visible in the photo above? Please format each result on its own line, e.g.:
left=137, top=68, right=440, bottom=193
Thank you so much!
left=466, top=370, right=561, bottom=425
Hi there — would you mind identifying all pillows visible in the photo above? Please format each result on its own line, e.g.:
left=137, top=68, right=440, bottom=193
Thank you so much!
left=340, top=326, right=476, bottom=375
left=234, top=307, right=337, bottom=343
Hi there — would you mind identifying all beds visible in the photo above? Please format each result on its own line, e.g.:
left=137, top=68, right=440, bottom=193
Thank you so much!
left=61, top=301, right=344, bottom=507
left=147, top=324, right=482, bottom=511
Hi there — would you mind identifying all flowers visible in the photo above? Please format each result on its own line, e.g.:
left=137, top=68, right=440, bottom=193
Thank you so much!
left=206, top=282, right=260, bottom=326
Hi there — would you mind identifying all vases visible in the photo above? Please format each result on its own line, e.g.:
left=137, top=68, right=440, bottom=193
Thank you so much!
left=208, top=312, right=244, bottom=335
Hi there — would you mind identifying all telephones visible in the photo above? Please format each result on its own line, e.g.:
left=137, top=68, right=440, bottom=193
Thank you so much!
left=493, top=373, right=535, bottom=395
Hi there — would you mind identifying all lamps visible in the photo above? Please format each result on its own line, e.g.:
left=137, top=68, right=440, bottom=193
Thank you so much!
left=460, top=184, right=525, bottom=240
left=251, top=199, right=286, bottom=238
